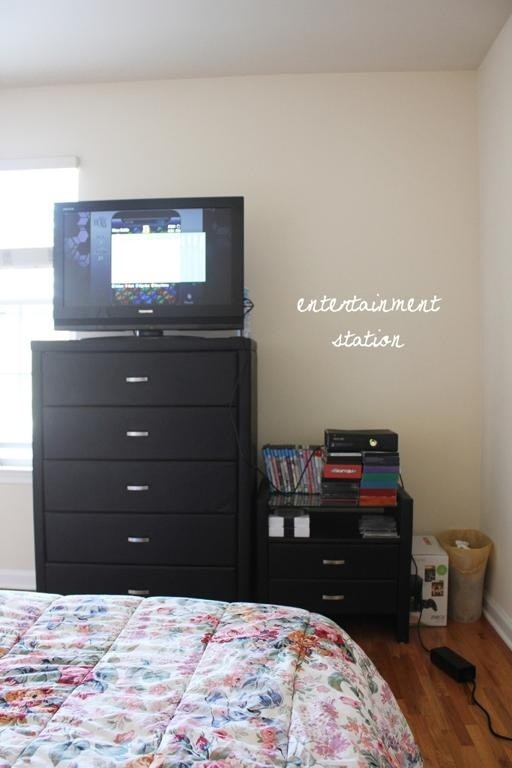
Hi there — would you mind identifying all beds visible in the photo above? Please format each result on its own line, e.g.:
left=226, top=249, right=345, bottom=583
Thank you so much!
left=0, top=590, right=424, bottom=767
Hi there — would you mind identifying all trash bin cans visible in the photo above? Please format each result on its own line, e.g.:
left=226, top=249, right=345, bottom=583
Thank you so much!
left=438, top=528, right=492, bottom=624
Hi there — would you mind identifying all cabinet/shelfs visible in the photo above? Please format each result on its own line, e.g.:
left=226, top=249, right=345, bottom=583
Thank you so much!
left=31, top=338, right=256, bottom=603
left=258, top=477, right=415, bottom=643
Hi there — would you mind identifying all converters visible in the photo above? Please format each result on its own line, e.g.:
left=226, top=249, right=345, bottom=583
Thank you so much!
left=430, top=646, right=477, bottom=683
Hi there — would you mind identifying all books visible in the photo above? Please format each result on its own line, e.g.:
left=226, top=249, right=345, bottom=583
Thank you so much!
left=258, top=442, right=324, bottom=496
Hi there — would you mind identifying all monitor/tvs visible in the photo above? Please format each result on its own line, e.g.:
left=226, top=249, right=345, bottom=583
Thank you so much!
left=53, top=197, right=246, bottom=341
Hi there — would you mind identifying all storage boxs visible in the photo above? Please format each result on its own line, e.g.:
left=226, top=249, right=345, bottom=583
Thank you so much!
left=410, top=535, right=450, bottom=629
